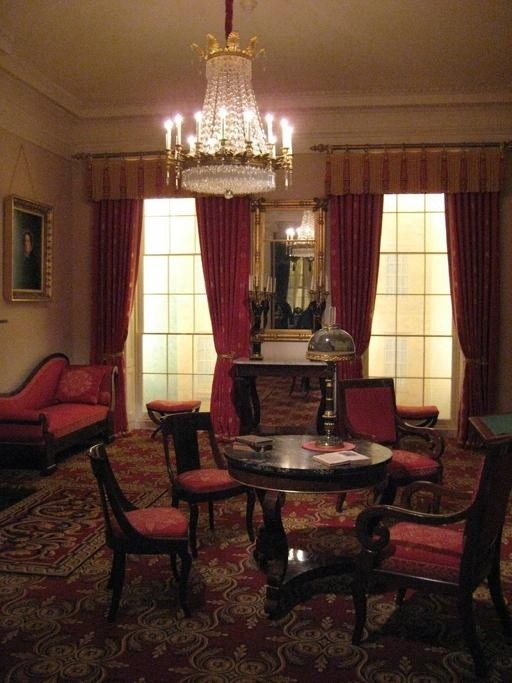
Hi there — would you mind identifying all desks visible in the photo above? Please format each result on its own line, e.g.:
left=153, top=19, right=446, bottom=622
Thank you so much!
left=228, top=357, right=334, bottom=435
left=223, top=434, right=393, bottom=614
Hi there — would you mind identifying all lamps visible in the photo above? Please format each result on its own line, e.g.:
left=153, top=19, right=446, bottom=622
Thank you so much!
left=305, top=323, right=358, bottom=449
left=282, top=209, right=316, bottom=274
left=163, top=1, right=295, bottom=200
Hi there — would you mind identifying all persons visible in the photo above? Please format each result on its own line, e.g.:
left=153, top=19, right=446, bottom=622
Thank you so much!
left=21, top=231, right=40, bottom=289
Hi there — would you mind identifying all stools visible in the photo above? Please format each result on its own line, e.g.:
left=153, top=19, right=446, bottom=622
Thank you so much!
left=146, top=400, right=200, bottom=438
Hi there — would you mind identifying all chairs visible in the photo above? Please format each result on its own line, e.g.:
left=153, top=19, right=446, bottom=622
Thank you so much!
left=337, top=377, right=444, bottom=512
left=161, top=412, right=256, bottom=560
left=350, top=443, right=512, bottom=683
left=88, top=443, right=192, bottom=621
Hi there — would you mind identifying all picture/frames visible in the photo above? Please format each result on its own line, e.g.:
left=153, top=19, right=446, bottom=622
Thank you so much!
left=2, top=194, right=57, bottom=307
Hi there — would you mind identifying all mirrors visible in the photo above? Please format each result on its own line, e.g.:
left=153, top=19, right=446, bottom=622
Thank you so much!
left=249, top=198, right=328, bottom=345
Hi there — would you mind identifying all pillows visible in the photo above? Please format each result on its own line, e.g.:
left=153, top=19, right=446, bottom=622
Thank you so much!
left=53, top=365, right=106, bottom=404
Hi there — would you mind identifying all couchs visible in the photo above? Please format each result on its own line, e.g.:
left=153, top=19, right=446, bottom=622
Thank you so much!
left=0, top=351, right=122, bottom=477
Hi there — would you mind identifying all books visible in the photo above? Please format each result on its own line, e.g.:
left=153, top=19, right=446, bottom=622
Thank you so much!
left=233, top=434, right=275, bottom=452
left=312, top=450, right=371, bottom=466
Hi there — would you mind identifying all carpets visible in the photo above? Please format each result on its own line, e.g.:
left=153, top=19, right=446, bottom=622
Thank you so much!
left=0, top=484, right=168, bottom=576
